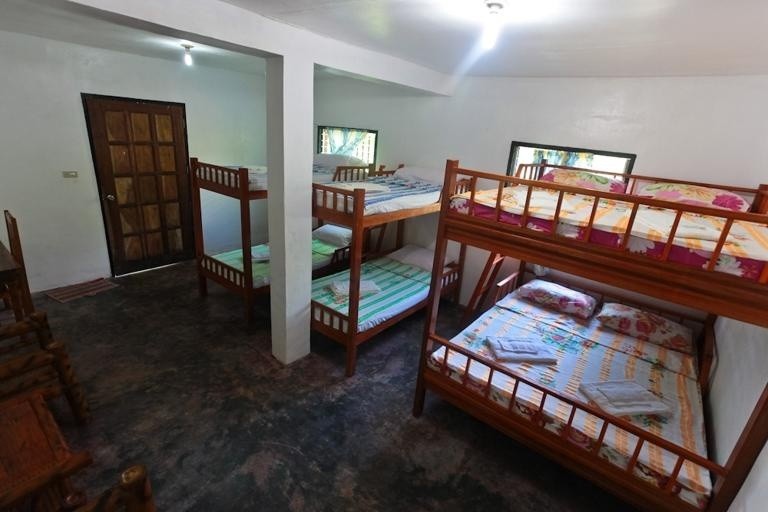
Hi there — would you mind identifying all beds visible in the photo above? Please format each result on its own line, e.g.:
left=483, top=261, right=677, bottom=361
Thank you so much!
left=189, top=157, right=375, bottom=333
left=312, top=163, right=473, bottom=376
left=413, top=159, right=768, bottom=512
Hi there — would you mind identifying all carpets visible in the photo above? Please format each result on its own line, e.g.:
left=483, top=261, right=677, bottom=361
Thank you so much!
left=41, top=276, right=120, bottom=304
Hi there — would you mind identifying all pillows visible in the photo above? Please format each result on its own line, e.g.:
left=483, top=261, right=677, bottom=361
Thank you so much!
left=533, top=167, right=755, bottom=217
left=515, top=279, right=695, bottom=354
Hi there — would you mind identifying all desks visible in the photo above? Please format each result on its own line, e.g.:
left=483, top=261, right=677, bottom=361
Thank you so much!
left=0, top=385, right=96, bottom=510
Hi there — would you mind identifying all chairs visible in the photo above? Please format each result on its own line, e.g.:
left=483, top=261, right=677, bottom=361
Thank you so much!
left=0, top=203, right=37, bottom=325
left=1, top=308, right=94, bottom=427
